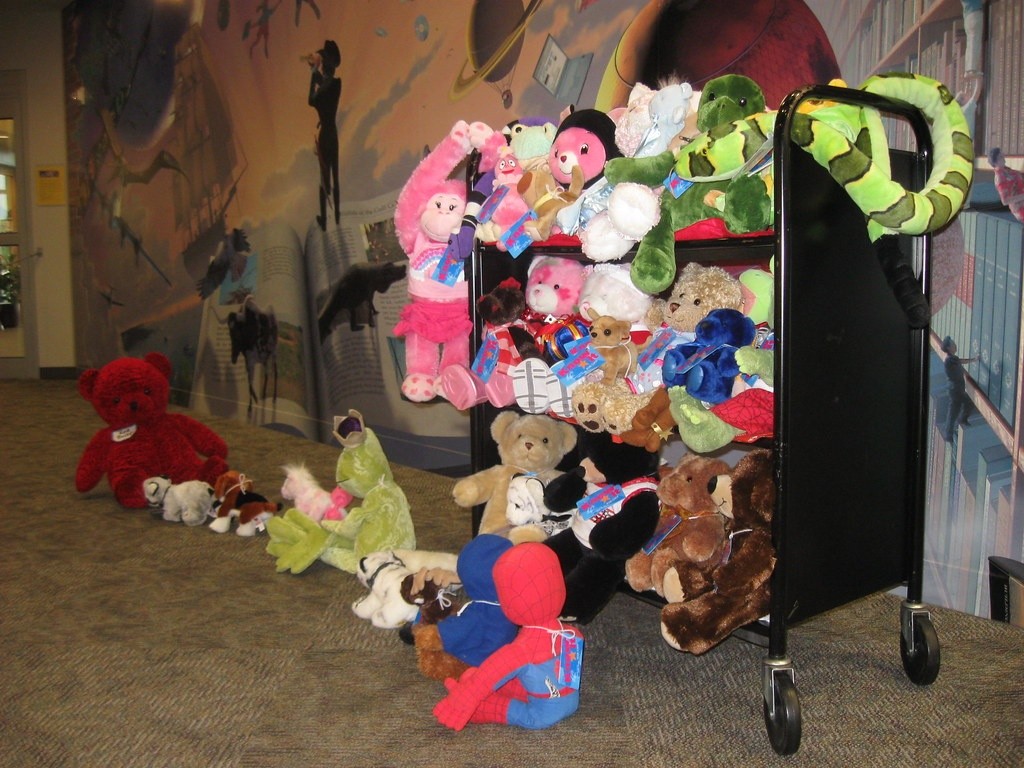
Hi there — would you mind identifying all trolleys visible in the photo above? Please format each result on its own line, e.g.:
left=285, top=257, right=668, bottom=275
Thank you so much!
left=465, top=82, right=942, bottom=756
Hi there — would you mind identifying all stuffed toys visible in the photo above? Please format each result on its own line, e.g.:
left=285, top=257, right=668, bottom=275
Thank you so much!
left=432, top=542, right=583, bottom=733
left=70, top=73, right=977, bottom=679
